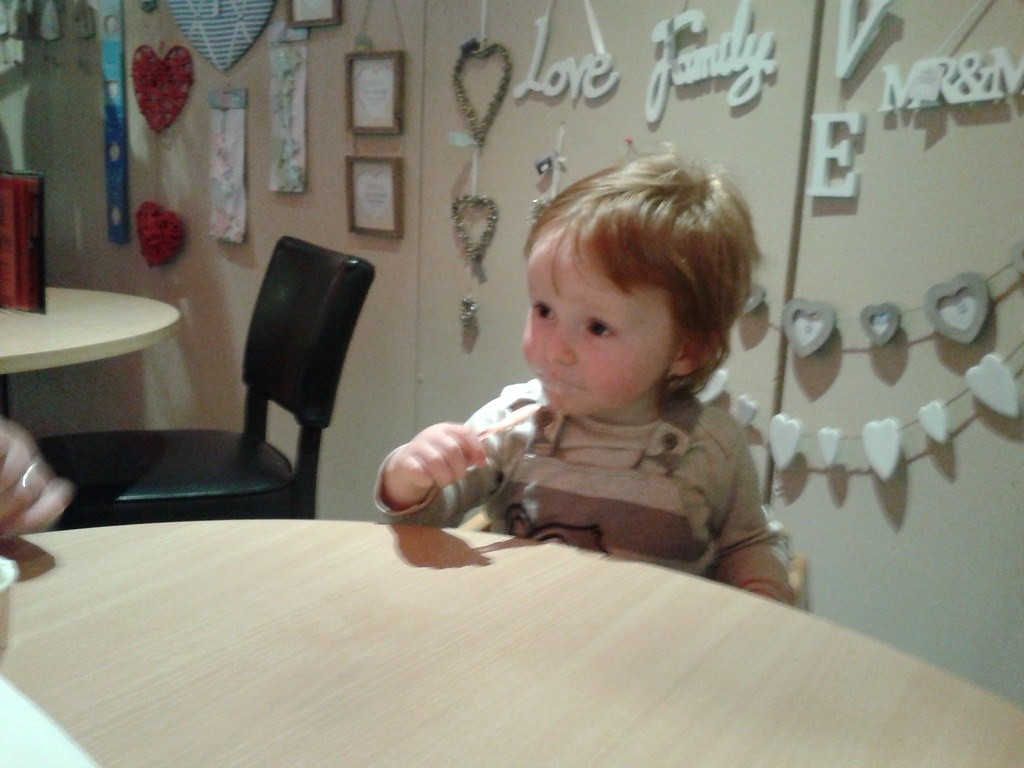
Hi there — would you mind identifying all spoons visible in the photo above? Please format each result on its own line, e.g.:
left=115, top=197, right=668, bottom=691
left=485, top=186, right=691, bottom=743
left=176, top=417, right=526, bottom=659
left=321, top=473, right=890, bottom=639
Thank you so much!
left=477, top=403, right=543, bottom=442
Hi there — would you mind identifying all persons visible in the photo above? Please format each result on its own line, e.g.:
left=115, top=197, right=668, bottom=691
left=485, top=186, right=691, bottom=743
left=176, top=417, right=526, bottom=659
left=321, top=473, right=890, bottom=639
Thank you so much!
left=0, top=418, right=73, bottom=535
left=374, top=154, right=798, bottom=603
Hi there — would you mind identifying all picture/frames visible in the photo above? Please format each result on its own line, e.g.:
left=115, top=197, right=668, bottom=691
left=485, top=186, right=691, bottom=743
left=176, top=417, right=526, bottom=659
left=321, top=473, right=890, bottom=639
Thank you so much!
left=344, top=51, right=402, bottom=134
left=286, top=0, right=339, bottom=28
left=346, top=156, right=404, bottom=239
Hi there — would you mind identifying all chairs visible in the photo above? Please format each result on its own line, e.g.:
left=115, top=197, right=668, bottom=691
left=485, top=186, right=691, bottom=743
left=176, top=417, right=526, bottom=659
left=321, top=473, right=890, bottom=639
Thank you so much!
left=37, top=235, right=375, bottom=532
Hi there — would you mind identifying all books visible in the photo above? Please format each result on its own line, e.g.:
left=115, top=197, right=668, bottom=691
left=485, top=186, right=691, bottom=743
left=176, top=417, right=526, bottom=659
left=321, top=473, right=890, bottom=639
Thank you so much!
left=0, top=172, right=46, bottom=315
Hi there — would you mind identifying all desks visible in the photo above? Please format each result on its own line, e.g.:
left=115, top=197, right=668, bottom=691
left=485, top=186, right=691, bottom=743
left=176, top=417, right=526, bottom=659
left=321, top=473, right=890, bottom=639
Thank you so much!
left=0, top=287, right=183, bottom=374
left=0, top=519, right=1024, bottom=768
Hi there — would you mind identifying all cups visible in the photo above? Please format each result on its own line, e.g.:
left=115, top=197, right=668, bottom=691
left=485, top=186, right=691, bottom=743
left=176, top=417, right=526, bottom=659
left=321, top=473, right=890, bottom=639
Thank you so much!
left=0, top=555, right=19, bottom=650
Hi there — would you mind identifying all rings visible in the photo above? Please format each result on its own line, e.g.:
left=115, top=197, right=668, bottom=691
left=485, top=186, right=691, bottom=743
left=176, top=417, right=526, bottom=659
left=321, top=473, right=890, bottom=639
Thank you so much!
left=20, top=463, right=48, bottom=504
